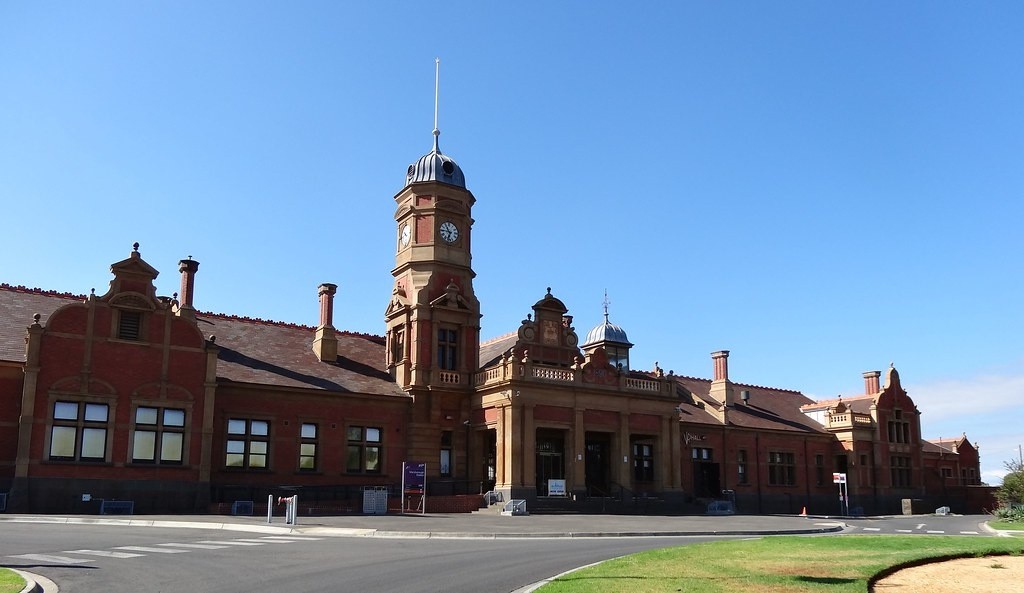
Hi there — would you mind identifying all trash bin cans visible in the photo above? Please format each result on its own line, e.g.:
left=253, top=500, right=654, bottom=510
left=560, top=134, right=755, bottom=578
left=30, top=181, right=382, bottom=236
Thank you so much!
left=360, top=486, right=376, bottom=515
left=374, top=486, right=388, bottom=517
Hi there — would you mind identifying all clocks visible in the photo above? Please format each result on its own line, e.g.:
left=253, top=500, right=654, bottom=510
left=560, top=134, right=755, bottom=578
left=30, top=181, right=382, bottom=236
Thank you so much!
left=439, top=221, right=459, bottom=243
left=400, top=224, right=411, bottom=246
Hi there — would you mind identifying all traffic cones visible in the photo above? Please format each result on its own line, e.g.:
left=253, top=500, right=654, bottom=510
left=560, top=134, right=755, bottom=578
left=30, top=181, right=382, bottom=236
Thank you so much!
left=799, top=507, right=807, bottom=517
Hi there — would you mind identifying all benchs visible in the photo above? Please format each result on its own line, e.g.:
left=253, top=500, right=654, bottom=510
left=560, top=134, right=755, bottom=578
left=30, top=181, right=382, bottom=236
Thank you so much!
left=99, top=500, right=134, bottom=515
